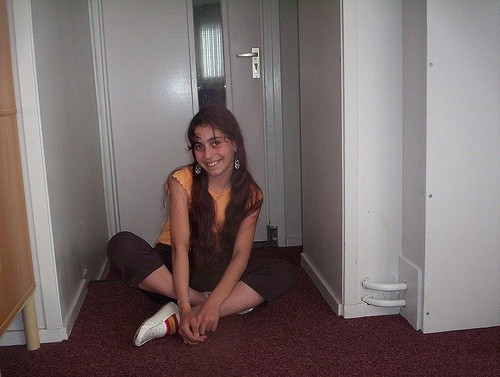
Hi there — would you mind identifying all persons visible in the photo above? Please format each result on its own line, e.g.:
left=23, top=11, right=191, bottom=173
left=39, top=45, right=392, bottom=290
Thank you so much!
left=107, top=106, right=296, bottom=347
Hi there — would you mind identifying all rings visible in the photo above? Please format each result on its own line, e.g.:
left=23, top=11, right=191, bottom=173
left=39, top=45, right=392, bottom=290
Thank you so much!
left=183, top=339, right=187, bottom=345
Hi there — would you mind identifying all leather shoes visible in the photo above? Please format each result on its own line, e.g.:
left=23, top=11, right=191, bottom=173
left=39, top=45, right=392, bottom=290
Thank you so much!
left=132, top=291, right=254, bottom=347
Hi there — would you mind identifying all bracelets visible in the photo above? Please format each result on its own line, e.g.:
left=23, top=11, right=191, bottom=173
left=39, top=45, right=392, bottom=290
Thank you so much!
left=177, top=297, right=190, bottom=304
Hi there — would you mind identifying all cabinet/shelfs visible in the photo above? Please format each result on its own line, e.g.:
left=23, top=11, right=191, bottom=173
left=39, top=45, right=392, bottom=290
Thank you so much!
left=0, top=0, right=40, bottom=351
